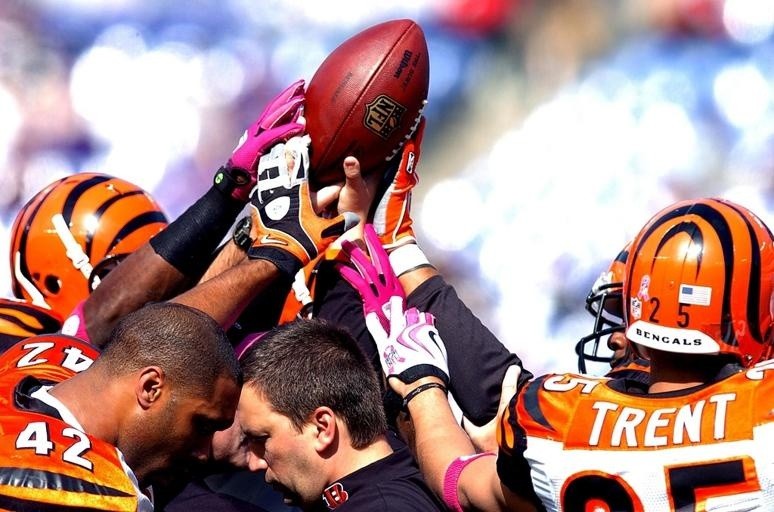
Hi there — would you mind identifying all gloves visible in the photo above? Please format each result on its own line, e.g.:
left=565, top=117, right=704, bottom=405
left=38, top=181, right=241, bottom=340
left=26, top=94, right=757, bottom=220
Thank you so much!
left=372, top=114, right=429, bottom=252
left=334, top=223, right=450, bottom=389
left=247, top=134, right=361, bottom=283
left=213, top=79, right=306, bottom=204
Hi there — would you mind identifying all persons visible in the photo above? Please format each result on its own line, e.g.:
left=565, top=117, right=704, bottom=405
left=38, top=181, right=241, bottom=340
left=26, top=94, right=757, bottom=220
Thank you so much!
left=1, top=80, right=774, bottom=512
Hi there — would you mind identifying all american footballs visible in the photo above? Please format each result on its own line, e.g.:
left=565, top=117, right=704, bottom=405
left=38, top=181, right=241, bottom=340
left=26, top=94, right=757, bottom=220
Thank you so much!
left=303, top=20, right=429, bottom=191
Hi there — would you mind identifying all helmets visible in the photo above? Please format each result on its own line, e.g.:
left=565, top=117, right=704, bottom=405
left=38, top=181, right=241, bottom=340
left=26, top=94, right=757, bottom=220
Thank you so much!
left=574, top=196, right=772, bottom=376
left=9, top=171, right=171, bottom=320
